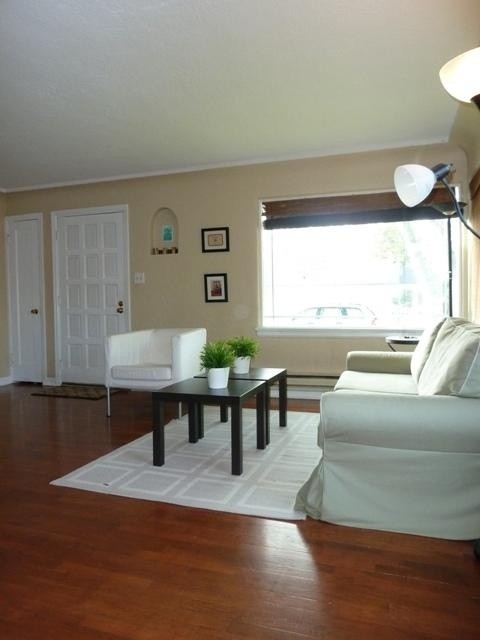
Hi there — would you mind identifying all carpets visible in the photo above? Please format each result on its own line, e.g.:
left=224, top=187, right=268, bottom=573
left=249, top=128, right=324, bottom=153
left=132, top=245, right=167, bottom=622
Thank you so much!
left=49, top=402, right=320, bottom=522
left=31, top=383, right=113, bottom=400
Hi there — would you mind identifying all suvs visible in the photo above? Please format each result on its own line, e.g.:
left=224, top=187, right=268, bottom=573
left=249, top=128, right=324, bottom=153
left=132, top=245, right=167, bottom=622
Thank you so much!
left=264, top=300, right=380, bottom=329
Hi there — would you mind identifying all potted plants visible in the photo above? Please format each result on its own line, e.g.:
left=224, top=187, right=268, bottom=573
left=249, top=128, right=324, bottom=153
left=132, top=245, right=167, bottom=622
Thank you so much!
left=200, top=336, right=263, bottom=390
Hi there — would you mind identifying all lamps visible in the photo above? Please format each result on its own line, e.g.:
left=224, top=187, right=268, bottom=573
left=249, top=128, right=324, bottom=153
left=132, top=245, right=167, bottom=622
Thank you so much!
left=393, top=45, right=480, bottom=240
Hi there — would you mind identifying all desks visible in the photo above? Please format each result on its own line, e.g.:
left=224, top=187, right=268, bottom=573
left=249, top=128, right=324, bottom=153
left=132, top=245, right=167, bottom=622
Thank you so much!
left=387, top=333, right=420, bottom=351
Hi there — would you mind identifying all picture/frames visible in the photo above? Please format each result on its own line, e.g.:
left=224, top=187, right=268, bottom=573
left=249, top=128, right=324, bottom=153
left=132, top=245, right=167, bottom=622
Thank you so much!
left=201, top=227, right=229, bottom=302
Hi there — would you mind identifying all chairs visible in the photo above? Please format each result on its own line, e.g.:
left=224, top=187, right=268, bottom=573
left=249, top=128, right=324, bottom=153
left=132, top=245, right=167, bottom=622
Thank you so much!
left=105, top=327, right=206, bottom=418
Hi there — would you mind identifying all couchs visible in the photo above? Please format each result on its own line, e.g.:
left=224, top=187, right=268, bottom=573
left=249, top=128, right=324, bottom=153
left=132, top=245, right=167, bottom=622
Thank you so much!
left=307, top=315, right=480, bottom=543
